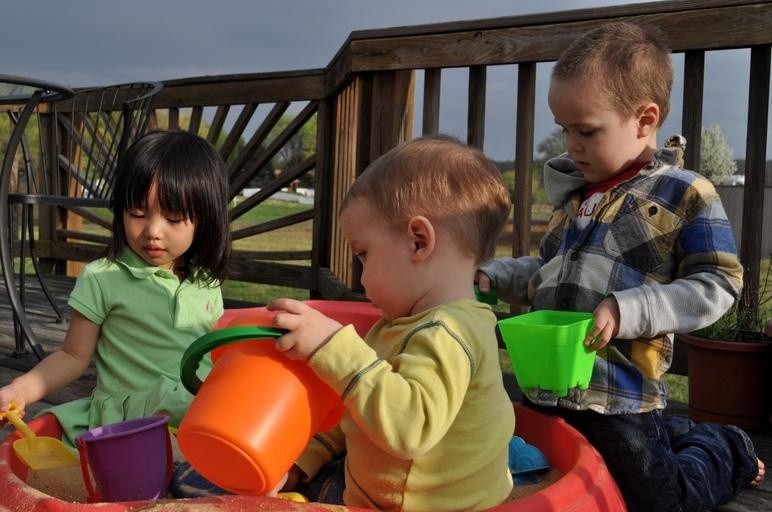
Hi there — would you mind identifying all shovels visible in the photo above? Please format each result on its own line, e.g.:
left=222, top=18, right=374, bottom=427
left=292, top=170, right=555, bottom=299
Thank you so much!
left=0, top=402, right=83, bottom=474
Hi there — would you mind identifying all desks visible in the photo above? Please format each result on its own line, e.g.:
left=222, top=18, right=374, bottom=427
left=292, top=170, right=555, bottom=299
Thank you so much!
left=1, top=74, right=70, bottom=362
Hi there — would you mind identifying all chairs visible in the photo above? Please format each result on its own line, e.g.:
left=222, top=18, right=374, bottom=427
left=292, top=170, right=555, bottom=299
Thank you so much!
left=1, top=73, right=164, bottom=379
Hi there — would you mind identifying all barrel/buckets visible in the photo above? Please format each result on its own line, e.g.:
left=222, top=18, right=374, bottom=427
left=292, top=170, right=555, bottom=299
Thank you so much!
left=75, top=415, right=174, bottom=503
left=180, top=327, right=347, bottom=497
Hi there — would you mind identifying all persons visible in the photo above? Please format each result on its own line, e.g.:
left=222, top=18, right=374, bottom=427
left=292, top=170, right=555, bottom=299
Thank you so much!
left=476, top=20, right=769, bottom=512
left=264, top=133, right=518, bottom=512
left=0, top=127, right=235, bottom=451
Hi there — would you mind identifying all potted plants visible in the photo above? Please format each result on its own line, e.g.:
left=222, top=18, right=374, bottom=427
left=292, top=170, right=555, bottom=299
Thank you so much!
left=677, top=256, right=771, bottom=431
